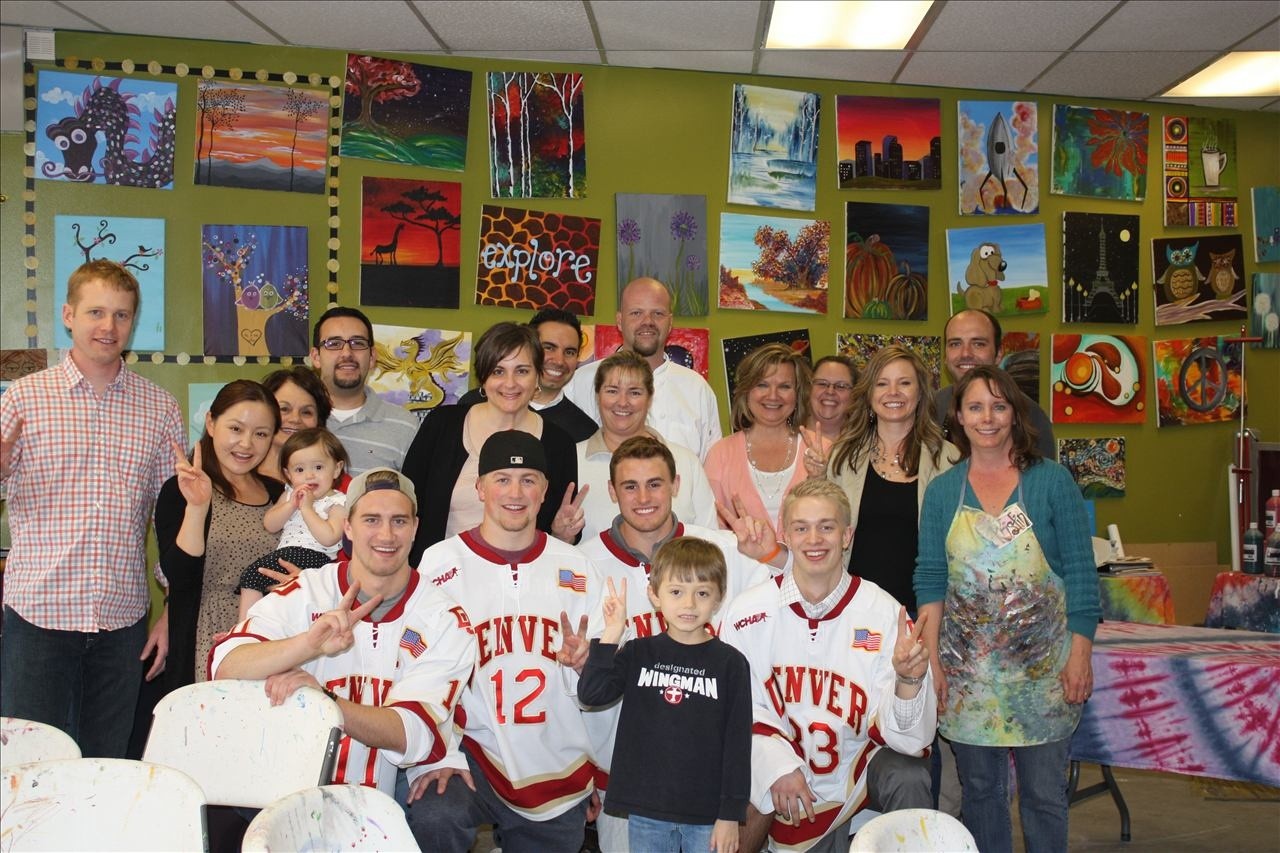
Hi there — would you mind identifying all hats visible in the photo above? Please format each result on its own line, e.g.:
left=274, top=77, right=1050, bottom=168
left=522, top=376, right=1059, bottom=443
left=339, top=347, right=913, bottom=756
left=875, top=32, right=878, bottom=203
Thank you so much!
left=478, top=429, right=548, bottom=491
left=342, top=465, right=418, bottom=557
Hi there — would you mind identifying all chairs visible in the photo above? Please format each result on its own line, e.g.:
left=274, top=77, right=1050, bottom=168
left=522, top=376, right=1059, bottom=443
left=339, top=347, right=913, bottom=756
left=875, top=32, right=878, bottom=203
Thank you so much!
left=0, top=677, right=422, bottom=853
left=848, top=808, right=980, bottom=853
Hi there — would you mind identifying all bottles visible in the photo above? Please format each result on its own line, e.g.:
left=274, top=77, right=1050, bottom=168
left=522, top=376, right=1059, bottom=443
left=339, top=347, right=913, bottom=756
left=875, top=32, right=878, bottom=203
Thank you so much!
left=1242, top=522, right=1264, bottom=573
left=1264, top=490, right=1280, bottom=540
left=1263, top=523, right=1280, bottom=577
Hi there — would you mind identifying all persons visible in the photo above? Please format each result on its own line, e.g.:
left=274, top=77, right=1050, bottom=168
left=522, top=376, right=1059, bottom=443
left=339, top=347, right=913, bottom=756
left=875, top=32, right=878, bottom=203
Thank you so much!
left=0, top=260, right=422, bottom=761
left=577, top=436, right=794, bottom=853
left=259, top=428, right=606, bottom=853
left=929, top=308, right=1056, bottom=461
left=912, top=365, right=1104, bottom=853
left=561, top=277, right=722, bottom=466
left=208, top=467, right=480, bottom=800
left=576, top=350, right=718, bottom=544
left=719, top=479, right=937, bottom=853
left=577, top=537, right=753, bottom=853
left=400, top=308, right=600, bottom=569
left=795, top=356, right=862, bottom=443
left=799, top=345, right=962, bottom=624
left=703, top=343, right=835, bottom=541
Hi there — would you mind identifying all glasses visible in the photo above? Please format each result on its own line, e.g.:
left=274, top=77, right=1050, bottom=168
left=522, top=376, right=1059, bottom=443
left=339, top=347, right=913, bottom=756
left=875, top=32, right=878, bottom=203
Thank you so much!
left=315, top=338, right=371, bottom=351
left=812, top=378, right=852, bottom=392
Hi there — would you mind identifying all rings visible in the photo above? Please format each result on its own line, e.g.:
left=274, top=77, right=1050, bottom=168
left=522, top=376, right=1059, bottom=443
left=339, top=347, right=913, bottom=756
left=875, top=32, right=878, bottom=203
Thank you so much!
left=804, top=447, right=810, bottom=456
left=1085, top=692, right=1088, bottom=697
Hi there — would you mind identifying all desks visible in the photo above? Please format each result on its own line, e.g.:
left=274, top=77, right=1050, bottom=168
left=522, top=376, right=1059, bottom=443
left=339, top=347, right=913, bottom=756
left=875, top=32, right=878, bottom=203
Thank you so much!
left=1007, top=621, right=1280, bottom=843
left=1096, top=572, right=1177, bottom=625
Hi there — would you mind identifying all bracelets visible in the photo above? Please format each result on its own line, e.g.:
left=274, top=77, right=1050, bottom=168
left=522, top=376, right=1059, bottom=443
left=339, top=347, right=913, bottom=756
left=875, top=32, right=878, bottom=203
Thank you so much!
left=322, top=686, right=338, bottom=702
left=163, top=596, right=170, bottom=608
left=897, top=672, right=926, bottom=684
left=758, top=544, right=781, bottom=563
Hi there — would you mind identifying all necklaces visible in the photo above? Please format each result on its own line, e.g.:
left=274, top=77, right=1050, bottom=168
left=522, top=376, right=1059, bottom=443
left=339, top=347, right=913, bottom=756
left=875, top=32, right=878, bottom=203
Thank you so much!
left=872, top=445, right=903, bottom=481
